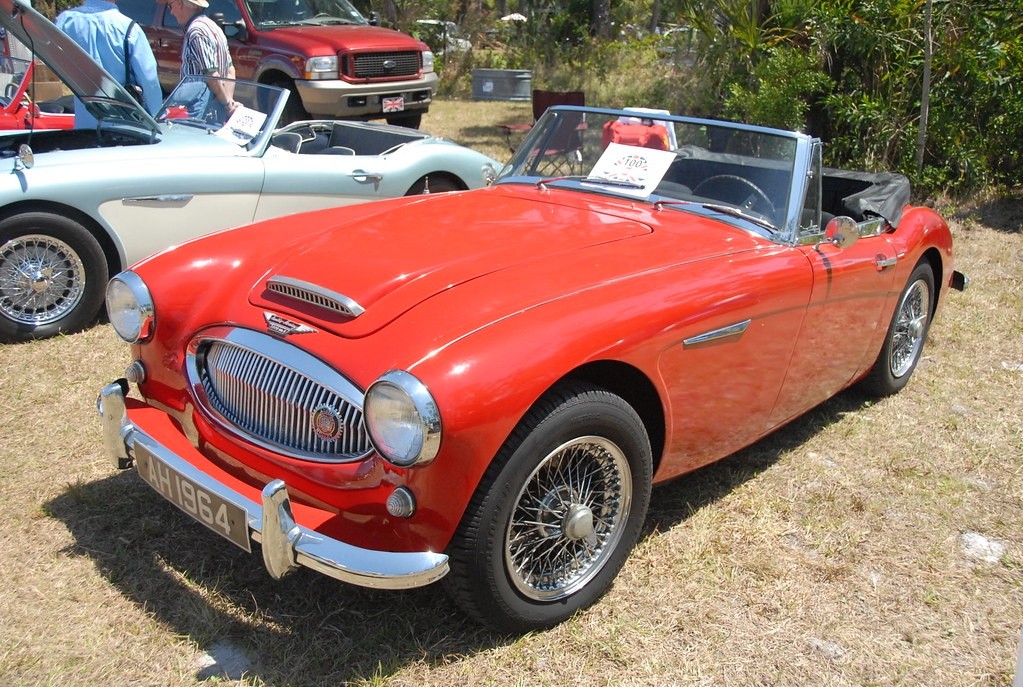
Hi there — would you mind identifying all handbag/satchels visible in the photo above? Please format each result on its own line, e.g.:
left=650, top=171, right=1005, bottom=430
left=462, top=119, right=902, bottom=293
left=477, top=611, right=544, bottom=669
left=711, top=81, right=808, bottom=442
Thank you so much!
left=124, top=84, right=151, bottom=121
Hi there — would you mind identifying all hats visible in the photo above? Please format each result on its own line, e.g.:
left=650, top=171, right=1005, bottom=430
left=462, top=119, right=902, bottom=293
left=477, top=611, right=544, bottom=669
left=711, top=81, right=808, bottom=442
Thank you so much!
left=157, top=0, right=209, bottom=8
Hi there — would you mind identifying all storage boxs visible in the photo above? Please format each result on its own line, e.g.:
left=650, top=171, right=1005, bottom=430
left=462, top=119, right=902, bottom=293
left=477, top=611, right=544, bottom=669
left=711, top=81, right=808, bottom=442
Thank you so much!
left=471, top=66, right=532, bottom=103
left=26, top=78, right=74, bottom=102
left=30, top=59, right=64, bottom=81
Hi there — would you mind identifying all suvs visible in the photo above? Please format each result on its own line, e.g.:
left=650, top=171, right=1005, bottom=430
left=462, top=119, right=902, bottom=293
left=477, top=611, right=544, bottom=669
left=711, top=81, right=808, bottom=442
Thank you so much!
left=112, top=0, right=439, bottom=128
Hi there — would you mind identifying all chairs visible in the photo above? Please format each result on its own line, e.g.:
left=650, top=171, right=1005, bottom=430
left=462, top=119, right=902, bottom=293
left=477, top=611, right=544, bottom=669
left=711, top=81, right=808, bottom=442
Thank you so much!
left=319, top=145, right=356, bottom=157
left=764, top=205, right=836, bottom=236
left=495, top=83, right=588, bottom=176
left=657, top=180, right=695, bottom=196
left=271, top=132, right=304, bottom=154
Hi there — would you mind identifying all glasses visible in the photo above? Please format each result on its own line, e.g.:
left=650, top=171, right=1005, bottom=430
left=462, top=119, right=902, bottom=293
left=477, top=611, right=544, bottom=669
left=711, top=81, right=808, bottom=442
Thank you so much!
left=167, top=1, right=176, bottom=10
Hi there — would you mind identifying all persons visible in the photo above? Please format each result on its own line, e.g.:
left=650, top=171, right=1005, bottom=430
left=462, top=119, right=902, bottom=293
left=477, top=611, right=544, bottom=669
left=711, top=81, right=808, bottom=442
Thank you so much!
left=54, top=0, right=163, bottom=128
left=163, top=0, right=245, bottom=127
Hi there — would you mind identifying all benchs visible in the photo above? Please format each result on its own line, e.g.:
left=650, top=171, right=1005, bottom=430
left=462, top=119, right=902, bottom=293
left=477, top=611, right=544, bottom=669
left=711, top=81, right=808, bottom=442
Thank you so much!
left=326, top=123, right=424, bottom=159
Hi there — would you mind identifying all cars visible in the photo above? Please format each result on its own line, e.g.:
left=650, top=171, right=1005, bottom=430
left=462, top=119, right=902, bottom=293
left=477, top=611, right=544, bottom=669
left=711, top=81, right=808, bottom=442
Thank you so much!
left=0, top=0, right=507, bottom=347
left=96, top=102, right=969, bottom=638
left=415, top=18, right=473, bottom=60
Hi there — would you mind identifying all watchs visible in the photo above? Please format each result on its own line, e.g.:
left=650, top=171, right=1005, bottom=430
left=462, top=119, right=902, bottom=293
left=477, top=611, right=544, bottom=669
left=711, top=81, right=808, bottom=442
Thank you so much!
left=221, top=100, right=230, bottom=106
